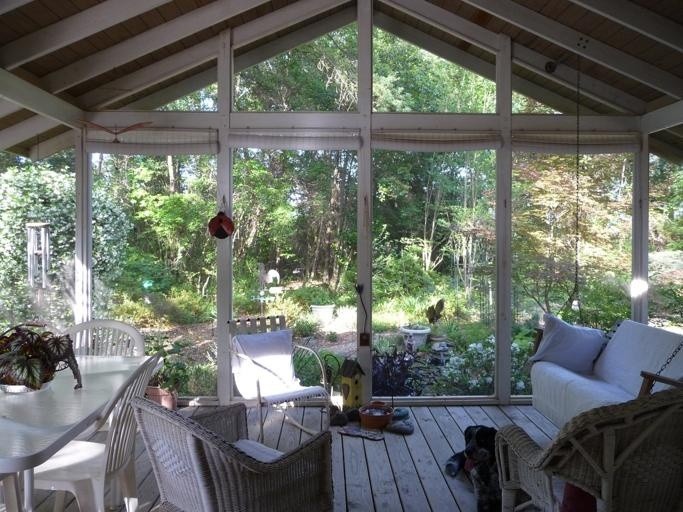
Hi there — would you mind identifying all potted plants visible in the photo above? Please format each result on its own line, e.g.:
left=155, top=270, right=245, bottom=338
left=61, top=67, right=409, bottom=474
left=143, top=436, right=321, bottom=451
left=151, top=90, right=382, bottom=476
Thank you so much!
left=0, top=325, right=83, bottom=398
left=145, top=361, right=186, bottom=411
left=399, top=295, right=430, bottom=351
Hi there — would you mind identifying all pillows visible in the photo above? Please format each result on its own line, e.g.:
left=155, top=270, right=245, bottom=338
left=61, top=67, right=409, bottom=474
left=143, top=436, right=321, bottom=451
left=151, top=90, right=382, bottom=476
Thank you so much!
left=232, top=330, right=299, bottom=397
left=529, top=312, right=605, bottom=375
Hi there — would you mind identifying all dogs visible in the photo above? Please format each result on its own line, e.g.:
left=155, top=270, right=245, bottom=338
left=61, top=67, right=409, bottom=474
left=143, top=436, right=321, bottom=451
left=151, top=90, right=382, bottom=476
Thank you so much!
left=445, top=425, right=497, bottom=477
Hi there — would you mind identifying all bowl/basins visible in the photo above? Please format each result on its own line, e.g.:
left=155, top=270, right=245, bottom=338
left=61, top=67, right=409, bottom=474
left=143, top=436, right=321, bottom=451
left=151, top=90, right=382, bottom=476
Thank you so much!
left=358, top=404, right=393, bottom=429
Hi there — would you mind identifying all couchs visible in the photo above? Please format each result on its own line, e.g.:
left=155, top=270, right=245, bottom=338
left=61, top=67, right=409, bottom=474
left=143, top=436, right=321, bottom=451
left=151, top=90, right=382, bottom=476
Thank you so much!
left=528, top=318, right=681, bottom=428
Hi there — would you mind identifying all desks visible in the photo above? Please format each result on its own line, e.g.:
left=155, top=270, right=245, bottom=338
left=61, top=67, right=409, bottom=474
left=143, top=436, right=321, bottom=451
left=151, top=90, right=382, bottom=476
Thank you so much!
left=0, top=354, right=164, bottom=475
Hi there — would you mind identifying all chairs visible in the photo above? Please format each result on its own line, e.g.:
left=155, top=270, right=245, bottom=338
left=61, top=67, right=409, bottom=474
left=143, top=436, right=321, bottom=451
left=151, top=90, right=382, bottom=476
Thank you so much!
left=60, top=319, right=145, bottom=357
left=227, top=314, right=328, bottom=444
left=31, top=352, right=161, bottom=512
left=131, top=397, right=334, bottom=512
left=492, top=386, right=680, bottom=512
